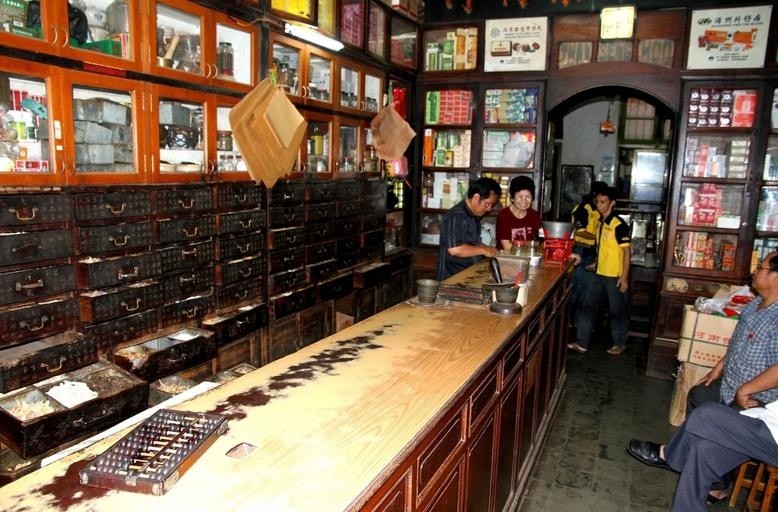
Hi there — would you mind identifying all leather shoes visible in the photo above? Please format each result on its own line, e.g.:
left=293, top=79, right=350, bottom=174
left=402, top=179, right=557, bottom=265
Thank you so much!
left=626, top=439, right=673, bottom=469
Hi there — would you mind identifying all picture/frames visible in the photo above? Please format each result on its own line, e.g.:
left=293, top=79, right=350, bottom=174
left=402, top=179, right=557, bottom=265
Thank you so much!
left=560, top=164, right=594, bottom=223
left=480, top=14, right=551, bottom=73
left=684, top=5, right=775, bottom=68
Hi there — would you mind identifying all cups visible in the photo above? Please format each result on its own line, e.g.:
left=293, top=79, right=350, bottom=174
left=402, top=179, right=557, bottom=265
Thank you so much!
left=500, top=239, right=538, bottom=256
left=415, top=277, right=439, bottom=306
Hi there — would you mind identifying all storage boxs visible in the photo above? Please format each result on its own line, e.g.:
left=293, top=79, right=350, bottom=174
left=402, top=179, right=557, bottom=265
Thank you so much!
left=668, top=362, right=715, bottom=426
left=678, top=305, right=740, bottom=367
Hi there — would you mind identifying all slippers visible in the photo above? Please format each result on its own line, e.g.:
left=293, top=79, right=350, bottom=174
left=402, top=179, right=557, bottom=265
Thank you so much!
left=568, top=342, right=585, bottom=353
left=607, top=343, right=625, bottom=355
left=704, top=491, right=727, bottom=506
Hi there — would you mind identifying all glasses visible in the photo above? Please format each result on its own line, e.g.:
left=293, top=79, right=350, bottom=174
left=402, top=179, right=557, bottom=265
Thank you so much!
left=757, top=265, right=774, bottom=272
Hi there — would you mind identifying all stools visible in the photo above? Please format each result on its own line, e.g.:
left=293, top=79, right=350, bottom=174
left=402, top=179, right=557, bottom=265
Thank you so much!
left=730, top=460, right=778, bottom=511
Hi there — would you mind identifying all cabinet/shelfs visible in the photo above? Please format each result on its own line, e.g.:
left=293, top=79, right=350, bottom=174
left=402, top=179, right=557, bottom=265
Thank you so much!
left=0, top=2, right=265, bottom=96
left=410, top=79, right=545, bottom=299
left=296, top=106, right=388, bottom=174
left=0, top=57, right=256, bottom=184
left=647, top=67, right=776, bottom=378
left=2, top=175, right=406, bottom=481
left=267, top=3, right=410, bottom=117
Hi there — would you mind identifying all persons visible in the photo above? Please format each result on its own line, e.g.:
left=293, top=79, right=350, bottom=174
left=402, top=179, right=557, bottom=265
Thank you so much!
left=567, top=182, right=608, bottom=328
left=495, top=176, right=544, bottom=250
left=627, top=400, right=778, bottom=512
left=566, top=188, right=631, bottom=356
left=685, top=251, right=778, bottom=507
left=437, top=177, right=501, bottom=281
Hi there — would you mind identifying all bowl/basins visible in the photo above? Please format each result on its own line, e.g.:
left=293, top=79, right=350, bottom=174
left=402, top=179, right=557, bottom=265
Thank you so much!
left=491, top=283, right=529, bottom=307
left=480, top=282, right=519, bottom=304
left=525, top=256, right=543, bottom=266
left=540, top=220, right=574, bottom=240
